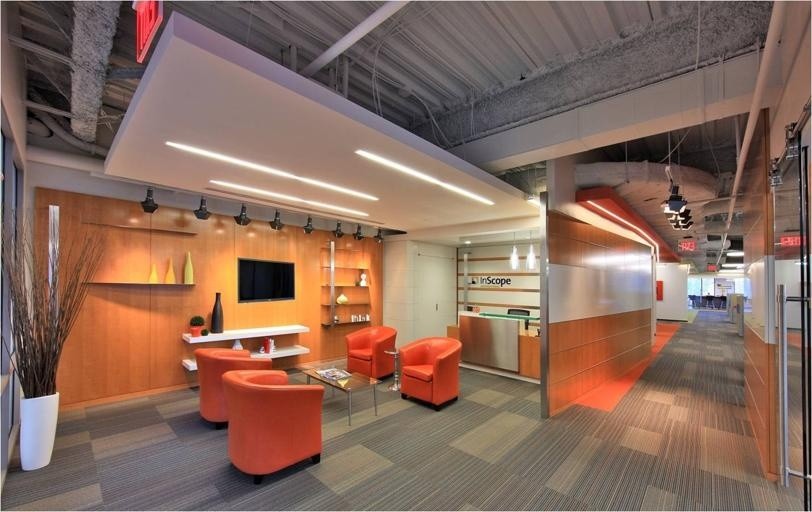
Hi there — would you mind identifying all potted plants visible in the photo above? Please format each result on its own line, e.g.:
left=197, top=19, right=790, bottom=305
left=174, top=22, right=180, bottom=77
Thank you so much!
left=1, top=217, right=108, bottom=472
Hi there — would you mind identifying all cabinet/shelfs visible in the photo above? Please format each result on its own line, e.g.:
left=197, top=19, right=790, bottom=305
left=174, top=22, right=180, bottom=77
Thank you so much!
left=319, top=246, right=371, bottom=328
left=80, top=221, right=197, bottom=287
left=182, top=325, right=310, bottom=371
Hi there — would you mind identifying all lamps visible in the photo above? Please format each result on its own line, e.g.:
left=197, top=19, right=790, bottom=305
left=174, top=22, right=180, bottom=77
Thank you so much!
left=510, top=232, right=518, bottom=269
left=526, top=231, right=536, bottom=269
left=660, top=179, right=694, bottom=231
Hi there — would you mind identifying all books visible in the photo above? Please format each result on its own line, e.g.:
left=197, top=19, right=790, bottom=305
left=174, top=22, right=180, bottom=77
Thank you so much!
left=323, top=369, right=352, bottom=381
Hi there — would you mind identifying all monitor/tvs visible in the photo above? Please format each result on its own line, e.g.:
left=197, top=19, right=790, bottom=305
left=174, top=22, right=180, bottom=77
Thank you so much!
left=238, top=258, right=295, bottom=303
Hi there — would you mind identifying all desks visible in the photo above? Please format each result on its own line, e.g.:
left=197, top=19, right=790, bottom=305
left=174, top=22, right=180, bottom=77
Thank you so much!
left=384, top=349, right=401, bottom=392
left=303, top=365, right=383, bottom=426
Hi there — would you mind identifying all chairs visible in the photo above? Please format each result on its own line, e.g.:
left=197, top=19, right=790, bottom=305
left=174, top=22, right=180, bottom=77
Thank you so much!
left=345, top=326, right=397, bottom=379
left=507, top=309, right=529, bottom=331
left=221, top=369, right=324, bottom=485
left=399, top=337, right=462, bottom=412
left=194, top=348, right=272, bottom=430
